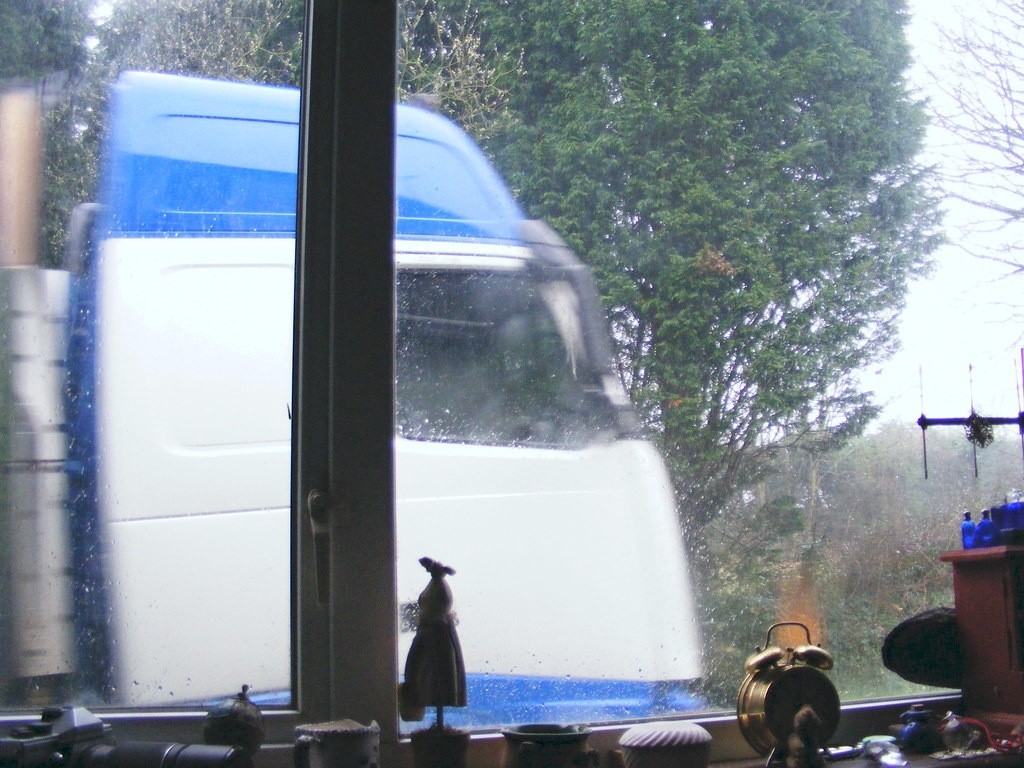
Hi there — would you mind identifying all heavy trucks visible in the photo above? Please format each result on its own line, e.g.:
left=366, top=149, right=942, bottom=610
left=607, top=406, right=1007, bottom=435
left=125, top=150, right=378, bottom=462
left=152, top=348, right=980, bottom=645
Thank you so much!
left=0, top=66, right=711, bottom=729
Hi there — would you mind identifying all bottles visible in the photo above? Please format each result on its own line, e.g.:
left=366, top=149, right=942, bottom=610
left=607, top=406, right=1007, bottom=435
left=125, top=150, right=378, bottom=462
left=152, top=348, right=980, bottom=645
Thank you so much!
left=960, top=510, right=977, bottom=548
left=973, top=510, right=1000, bottom=545
left=898, top=703, right=943, bottom=753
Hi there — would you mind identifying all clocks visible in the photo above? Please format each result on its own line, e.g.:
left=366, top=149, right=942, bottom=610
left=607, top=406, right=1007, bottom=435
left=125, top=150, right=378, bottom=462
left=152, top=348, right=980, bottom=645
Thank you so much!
left=737, top=622, right=841, bottom=768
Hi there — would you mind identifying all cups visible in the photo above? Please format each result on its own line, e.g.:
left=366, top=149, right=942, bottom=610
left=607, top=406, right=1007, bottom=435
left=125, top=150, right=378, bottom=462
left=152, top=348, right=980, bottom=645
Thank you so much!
left=500, top=723, right=596, bottom=767
left=294, top=722, right=381, bottom=768
